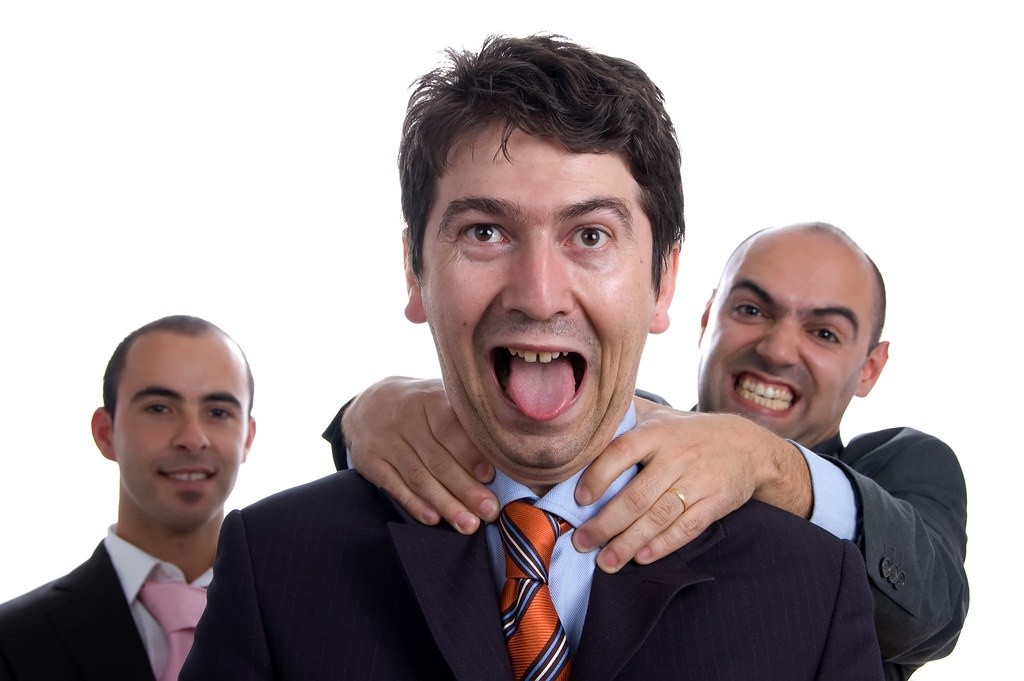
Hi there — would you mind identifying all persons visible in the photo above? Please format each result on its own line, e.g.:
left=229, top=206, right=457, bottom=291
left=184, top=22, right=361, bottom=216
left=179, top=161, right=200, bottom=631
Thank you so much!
left=0, top=313, right=256, bottom=681
left=321, top=222, right=970, bottom=681
left=177, top=32, right=884, bottom=681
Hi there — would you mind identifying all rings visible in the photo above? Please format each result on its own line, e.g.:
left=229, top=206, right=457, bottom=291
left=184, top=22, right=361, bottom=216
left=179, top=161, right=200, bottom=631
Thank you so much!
left=668, top=489, right=687, bottom=512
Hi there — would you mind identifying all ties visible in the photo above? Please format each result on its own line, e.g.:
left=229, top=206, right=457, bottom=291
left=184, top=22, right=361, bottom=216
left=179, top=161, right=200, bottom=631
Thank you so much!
left=137, top=577, right=208, bottom=681
left=486, top=495, right=577, bottom=681
